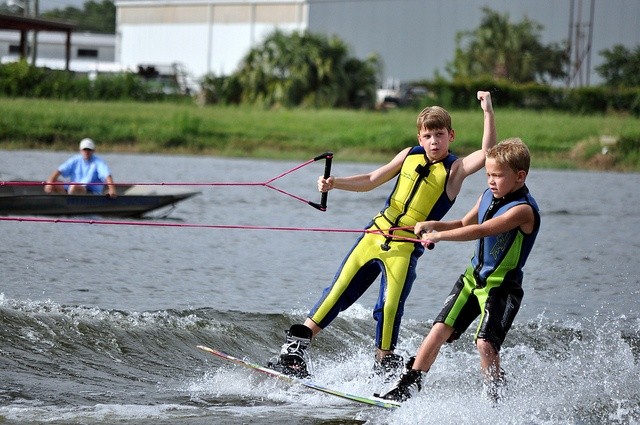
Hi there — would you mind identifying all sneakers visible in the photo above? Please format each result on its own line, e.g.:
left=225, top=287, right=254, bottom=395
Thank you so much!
left=278, top=323, right=312, bottom=378
left=378, top=356, right=430, bottom=400
left=367, top=354, right=404, bottom=385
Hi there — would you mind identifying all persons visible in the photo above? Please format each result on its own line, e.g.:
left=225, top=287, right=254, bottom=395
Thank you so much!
left=277, top=88, right=498, bottom=389
left=44, top=137, right=118, bottom=199
left=374, top=136, right=541, bottom=407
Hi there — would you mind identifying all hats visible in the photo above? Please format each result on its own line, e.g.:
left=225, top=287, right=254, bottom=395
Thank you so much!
left=79, top=137, right=95, bottom=151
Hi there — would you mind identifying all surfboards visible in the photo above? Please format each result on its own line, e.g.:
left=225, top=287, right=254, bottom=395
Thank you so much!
left=197, top=343, right=401, bottom=410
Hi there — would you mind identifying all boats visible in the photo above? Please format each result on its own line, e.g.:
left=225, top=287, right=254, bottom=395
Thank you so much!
left=0, top=180, right=201, bottom=218
